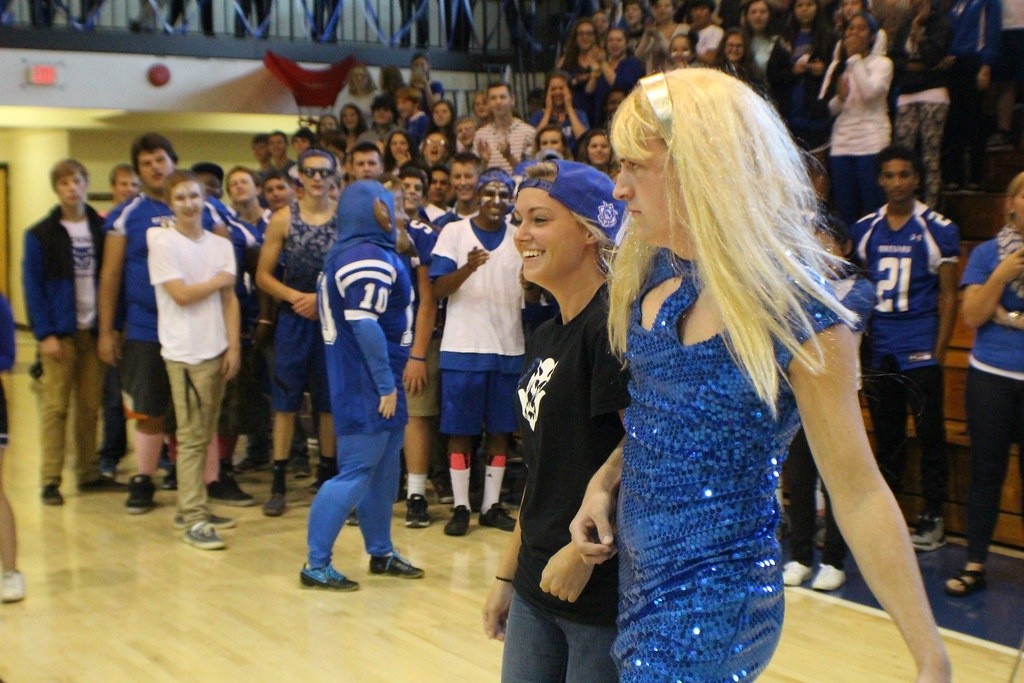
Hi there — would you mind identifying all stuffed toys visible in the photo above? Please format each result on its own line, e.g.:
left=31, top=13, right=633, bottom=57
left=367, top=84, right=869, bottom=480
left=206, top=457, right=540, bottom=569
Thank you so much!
left=297, top=179, right=423, bottom=593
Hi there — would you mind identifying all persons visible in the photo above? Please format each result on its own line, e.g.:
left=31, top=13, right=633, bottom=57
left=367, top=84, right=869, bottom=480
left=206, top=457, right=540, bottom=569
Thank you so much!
left=98, top=131, right=254, bottom=516
left=567, top=68, right=957, bottom=681
left=427, top=167, right=528, bottom=540
left=839, top=144, right=957, bottom=551
left=479, top=152, right=627, bottom=683
left=0, top=0, right=1024, bottom=682
left=256, top=147, right=337, bottom=518
left=941, top=173, right=1024, bottom=594
left=21, top=160, right=123, bottom=506
left=143, top=168, right=243, bottom=551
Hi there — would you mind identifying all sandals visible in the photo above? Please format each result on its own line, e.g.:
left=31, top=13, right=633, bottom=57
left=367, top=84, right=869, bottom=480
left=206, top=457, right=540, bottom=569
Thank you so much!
left=944, top=568, right=986, bottom=597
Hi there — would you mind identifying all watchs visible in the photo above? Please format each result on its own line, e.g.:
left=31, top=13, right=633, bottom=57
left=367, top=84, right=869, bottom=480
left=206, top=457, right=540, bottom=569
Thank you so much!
left=1005, top=310, right=1020, bottom=329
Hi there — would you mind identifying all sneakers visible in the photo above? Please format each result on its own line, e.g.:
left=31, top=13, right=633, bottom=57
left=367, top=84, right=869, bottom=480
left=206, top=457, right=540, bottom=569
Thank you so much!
left=811, top=562, right=846, bottom=590
left=782, top=561, right=814, bottom=586
left=370, top=548, right=424, bottom=579
left=910, top=513, right=948, bottom=552
left=300, top=562, right=360, bottom=592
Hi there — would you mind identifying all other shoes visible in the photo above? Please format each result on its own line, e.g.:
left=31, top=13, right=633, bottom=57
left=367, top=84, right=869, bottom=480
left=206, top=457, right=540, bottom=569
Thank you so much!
left=42, top=484, right=65, bottom=505
left=0, top=571, right=24, bottom=602
left=73, top=432, right=527, bottom=550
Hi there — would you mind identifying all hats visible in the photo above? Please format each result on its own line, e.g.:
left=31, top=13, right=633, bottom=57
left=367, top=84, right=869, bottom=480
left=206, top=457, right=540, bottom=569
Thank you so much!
left=517, top=159, right=626, bottom=240
left=191, top=162, right=225, bottom=184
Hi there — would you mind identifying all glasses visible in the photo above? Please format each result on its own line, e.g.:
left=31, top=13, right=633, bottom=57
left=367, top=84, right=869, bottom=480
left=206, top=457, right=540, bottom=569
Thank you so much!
left=424, top=137, right=450, bottom=148
left=483, top=188, right=513, bottom=201
left=303, top=167, right=333, bottom=181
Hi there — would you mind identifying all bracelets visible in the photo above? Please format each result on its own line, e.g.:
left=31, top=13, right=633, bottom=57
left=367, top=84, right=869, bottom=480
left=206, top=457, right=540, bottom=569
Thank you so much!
left=495, top=576, right=513, bottom=583
left=257, top=319, right=274, bottom=325
left=406, top=354, right=428, bottom=363
